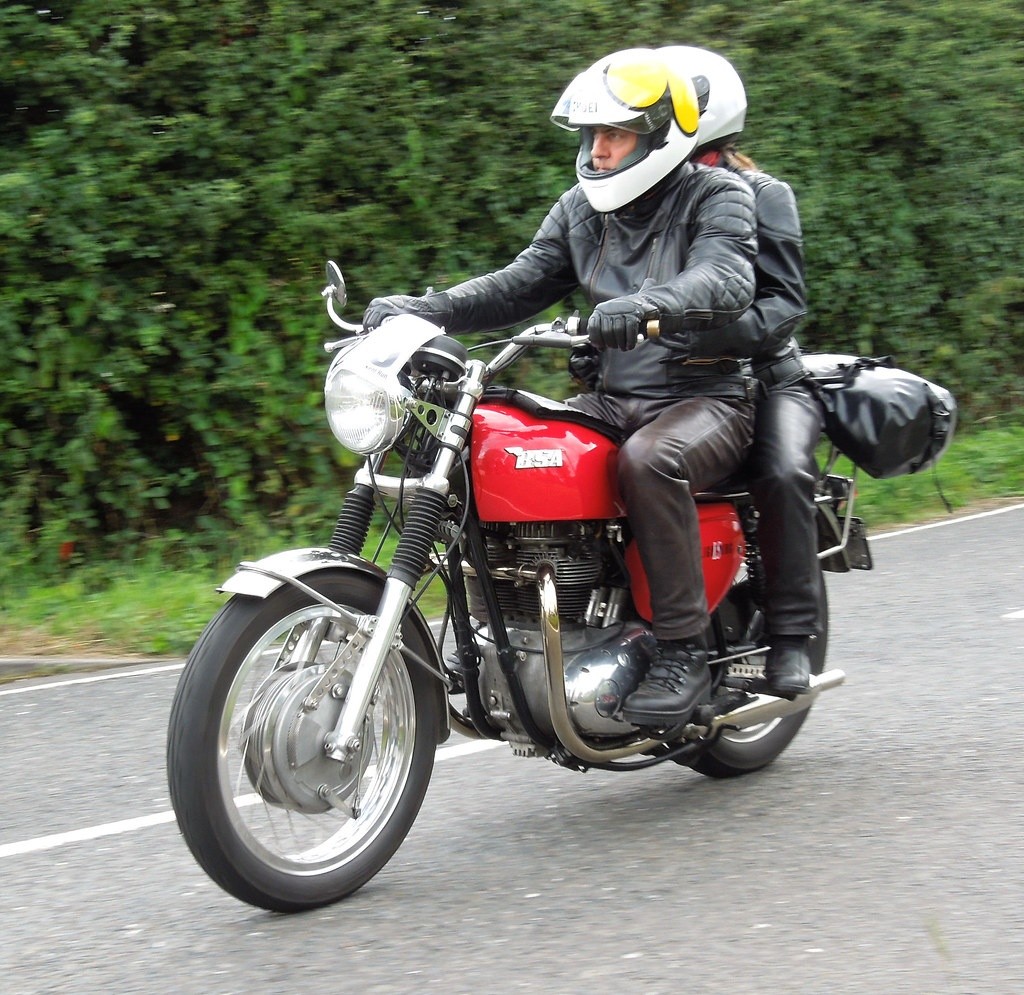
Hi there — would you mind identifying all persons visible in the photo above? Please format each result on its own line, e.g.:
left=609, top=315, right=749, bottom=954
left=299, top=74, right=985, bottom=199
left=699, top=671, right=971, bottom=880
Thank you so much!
left=363, top=46, right=825, bottom=732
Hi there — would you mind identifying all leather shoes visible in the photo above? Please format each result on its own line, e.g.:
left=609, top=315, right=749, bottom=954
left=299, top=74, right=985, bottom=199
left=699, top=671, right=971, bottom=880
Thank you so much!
left=622, top=633, right=713, bottom=725
left=764, top=633, right=810, bottom=693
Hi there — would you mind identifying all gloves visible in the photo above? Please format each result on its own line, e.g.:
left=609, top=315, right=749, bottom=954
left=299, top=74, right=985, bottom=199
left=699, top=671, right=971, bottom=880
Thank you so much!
left=656, top=330, right=692, bottom=365
left=570, top=349, right=599, bottom=391
left=590, top=286, right=681, bottom=351
left=362, top=286, right=452, bottom=335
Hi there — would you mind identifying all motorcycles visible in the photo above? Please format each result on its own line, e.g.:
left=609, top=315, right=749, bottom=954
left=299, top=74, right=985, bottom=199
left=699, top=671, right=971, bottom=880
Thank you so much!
left=166, top=259, right=876, bottom=914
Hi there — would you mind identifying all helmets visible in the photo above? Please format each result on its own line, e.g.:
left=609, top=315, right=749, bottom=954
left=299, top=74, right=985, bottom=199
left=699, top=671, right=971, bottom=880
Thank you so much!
left=656, top=44, right=745, bottom=146
left=567, top=46, right=700, bottom=212
